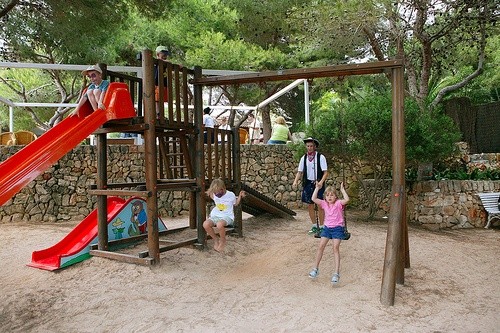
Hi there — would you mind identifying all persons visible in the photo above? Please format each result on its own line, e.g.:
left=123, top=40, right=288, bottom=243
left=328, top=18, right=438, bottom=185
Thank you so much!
left=267, top=117, right=293, bottom=144
left=203, top=107, right=231, bottom=144
left=153, top=46, right=171, bottom=120
left=68, top=65, right=109, bottom=118
left=309, top=181, right=350, bottom=284
left=203, top=179, right=246, bottom=251
left=292, top=137, right=328, bottom=234
left=248, top=114, right=260, bottom=144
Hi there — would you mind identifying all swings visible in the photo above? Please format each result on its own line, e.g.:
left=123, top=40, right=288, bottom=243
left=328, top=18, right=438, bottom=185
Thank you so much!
left=307, top=73, right=346, bottom=240
left=212, top=80, right=264, bottom=228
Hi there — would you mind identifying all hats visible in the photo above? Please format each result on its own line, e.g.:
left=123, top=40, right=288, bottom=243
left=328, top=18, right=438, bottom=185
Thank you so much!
left=156, top=46, right=170, bottom=55
left=303, top=137, right=319, bottom=148
left=81, top=65, right=102, bottom=76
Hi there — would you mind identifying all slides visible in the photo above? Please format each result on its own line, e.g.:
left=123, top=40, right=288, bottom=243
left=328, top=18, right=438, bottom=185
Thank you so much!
left=26, top=196, right=167, bottom=271
left=0, top=82, right=137, bottom=207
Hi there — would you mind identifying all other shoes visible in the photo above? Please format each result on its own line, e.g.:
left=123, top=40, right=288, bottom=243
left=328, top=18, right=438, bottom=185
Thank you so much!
left=309, top=224, right=324, bottom=235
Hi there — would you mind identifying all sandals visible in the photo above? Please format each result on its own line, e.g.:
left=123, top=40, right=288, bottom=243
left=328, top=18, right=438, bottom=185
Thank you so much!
left=331, top=273, right=340, bottom=284
left=309, top=268, right=319, bottom=278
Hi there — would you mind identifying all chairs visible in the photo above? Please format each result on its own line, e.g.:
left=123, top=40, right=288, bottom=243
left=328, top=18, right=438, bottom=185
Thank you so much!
left=12, top=130, right=37, bottom=145
left=0, top=132, right=13, bottom=145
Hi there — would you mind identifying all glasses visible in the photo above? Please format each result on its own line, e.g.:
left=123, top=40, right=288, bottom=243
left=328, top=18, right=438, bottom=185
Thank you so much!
left=88, top=73, right=96, bottom=78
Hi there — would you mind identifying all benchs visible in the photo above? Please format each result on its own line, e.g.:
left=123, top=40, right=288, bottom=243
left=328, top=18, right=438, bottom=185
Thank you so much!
left=107, top=139, right=134, bottom=144
left=475, top=192, right=500, bottom=229
